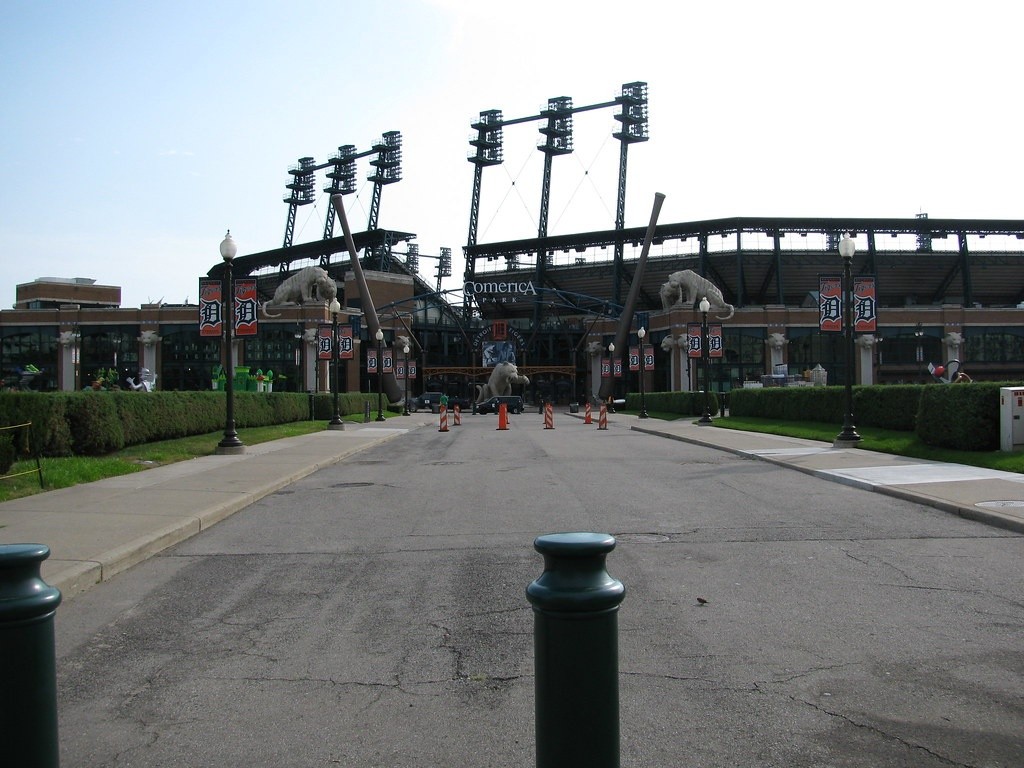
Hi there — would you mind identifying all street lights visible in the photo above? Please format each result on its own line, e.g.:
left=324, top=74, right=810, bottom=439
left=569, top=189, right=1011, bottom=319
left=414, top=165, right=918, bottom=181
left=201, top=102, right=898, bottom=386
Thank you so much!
left=608, top=342, right=616, bottom=413
left=699, top=297, right=713, bottom=422
left=638, top=327, right=649, bottom=418
left=218, top=229, right=243, bottom=445
left=375, top=328, right=387, bottom=422
left=328, top=297, right=344, bottom=424
left=403, top=345, right=411, bottom=416
left=836, top=233, right=861, bottom=441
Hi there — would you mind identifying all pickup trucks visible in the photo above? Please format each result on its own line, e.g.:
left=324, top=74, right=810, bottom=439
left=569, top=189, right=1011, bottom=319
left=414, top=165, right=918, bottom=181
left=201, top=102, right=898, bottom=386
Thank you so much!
left=408, top=392, right=470, bottom=413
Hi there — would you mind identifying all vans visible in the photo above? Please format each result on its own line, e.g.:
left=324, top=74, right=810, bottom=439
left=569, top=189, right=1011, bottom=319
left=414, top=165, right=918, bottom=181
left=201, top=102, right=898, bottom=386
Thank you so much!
left=476, top=396, right=524, bottom=415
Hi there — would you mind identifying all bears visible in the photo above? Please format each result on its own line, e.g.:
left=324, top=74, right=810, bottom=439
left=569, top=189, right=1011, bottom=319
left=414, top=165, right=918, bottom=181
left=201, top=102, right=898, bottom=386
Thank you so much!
left=660, top=282, right=682, bottom=309
left=316, top=276, right=338, bottom=301
